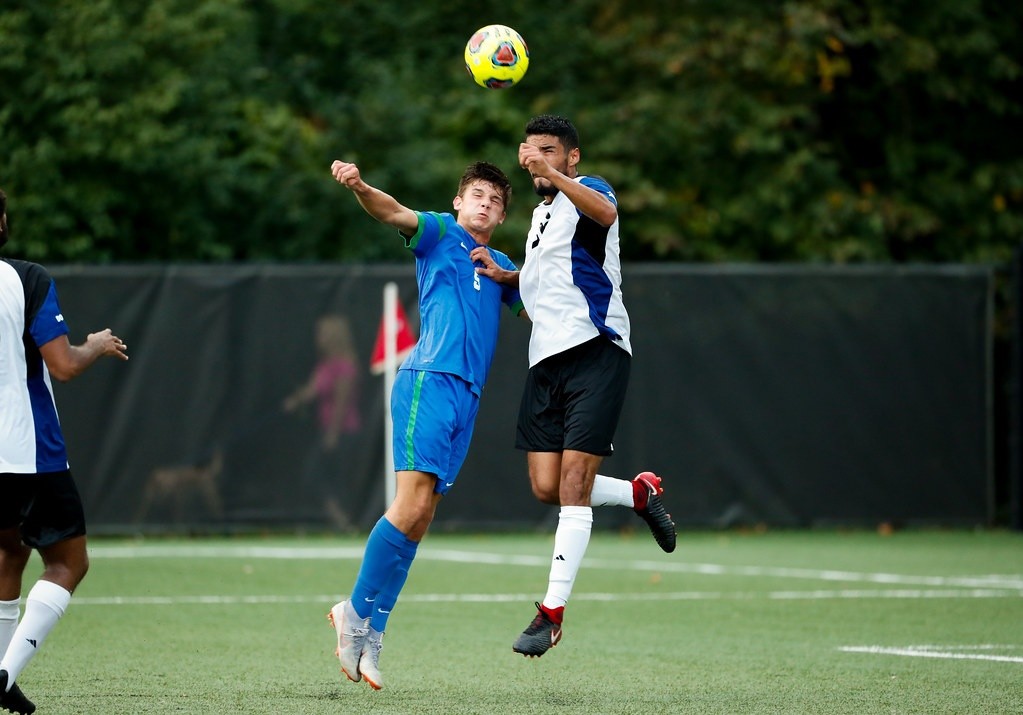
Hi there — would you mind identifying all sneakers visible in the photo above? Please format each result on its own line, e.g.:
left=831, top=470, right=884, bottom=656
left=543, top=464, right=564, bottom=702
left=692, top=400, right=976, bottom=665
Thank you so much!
left=327, top=599, right=372, bottom=682
left=0, top=669, right=35, bottom=715
left=358, top=625, right=385, bottom=690
left=513, top=601, right=562, bottom=658
left=633, top=472, right=678, bottom=553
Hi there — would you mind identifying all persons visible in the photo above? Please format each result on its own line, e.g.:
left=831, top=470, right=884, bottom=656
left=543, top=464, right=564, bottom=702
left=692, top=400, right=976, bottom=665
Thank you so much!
left=0, top=192, right=128, bottom=714
left=471, top=115, right=678, bottom=657
left=328, top=160, right=525, bottom=690
left=281, top=312, right=363, bottom=535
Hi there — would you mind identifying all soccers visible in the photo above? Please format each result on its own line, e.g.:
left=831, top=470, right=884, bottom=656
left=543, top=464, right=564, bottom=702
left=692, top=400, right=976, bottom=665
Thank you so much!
left=463, top=24, right=532, bottom=92
